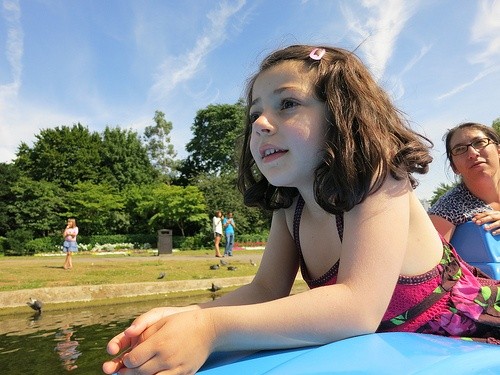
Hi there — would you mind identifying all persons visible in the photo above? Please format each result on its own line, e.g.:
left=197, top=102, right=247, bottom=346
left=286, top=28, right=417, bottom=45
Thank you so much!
left=61, top=217, right=79, bottom=271
left=103, top=44, right=500, bottom=375
left=426, top=122, right=500, bottom=243
left=212, top=209, right=235, bottom=258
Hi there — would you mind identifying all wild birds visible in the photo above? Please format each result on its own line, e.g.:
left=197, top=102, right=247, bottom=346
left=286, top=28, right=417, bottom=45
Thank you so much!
left=25, top=297, right=43, bottom=313
left=220, top=260, right=228, bottom=265
left=156, top=272, right=167, bottom=280
left=210, top=264, right=220, bottom=270
left=249, top=258, right=257, bottom=267
left=207, top=283, right=221, bottom=292
left=228, top=266, right=239, bottom=270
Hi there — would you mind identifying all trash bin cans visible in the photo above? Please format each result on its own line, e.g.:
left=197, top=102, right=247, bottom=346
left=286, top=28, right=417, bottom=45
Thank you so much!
left=158, top=229, right=172, bottom=254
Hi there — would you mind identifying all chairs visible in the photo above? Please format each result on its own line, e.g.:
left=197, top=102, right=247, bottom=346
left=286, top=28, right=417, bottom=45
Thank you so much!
left=450, top=220, right=500, bottom=279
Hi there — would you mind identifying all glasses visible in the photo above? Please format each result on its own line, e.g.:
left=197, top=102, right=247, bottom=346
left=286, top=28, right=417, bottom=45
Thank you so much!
left=449, top=138, right=498, bottom=156
